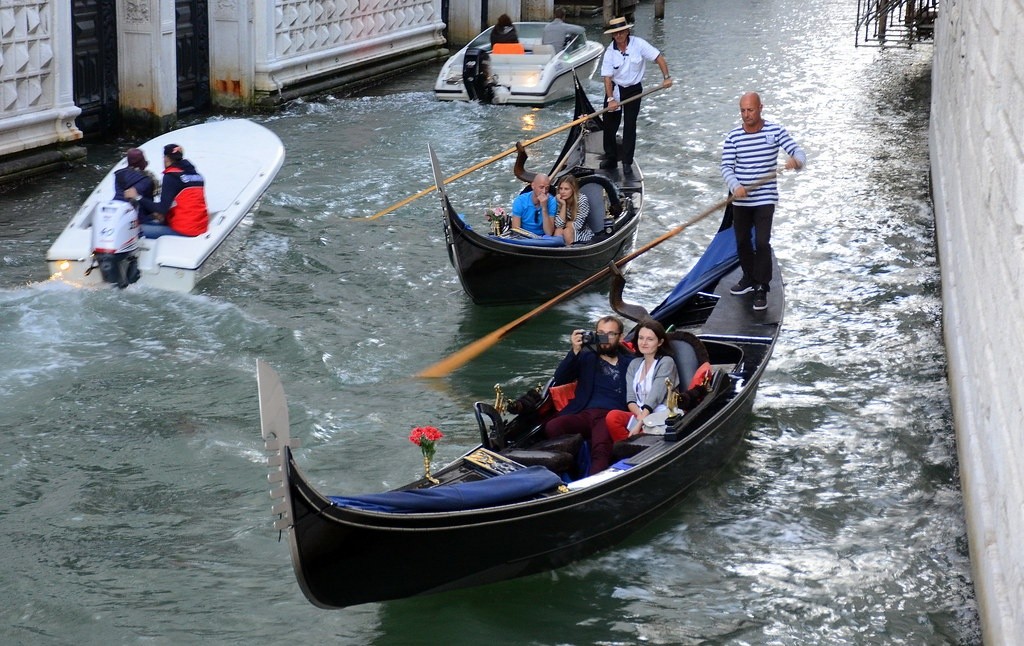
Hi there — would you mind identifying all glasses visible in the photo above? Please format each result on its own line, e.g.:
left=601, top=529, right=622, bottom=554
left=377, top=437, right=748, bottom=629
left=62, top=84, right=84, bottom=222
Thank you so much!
left=596, top=330, right=621, bottom=338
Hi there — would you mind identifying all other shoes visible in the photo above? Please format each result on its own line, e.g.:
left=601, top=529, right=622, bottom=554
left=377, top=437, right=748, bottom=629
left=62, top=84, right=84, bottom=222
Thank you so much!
left=623, top=163, right=633, bottom=175
left=600, top=159, right=617, bottom=169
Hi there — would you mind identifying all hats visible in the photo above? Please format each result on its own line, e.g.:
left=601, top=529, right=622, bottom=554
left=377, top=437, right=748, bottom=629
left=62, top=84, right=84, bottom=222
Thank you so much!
left=604, top=17, right=635, bottom=35
left=127, top=148, right=144, bottom=165
left=164, top=144, right=182, bottom=160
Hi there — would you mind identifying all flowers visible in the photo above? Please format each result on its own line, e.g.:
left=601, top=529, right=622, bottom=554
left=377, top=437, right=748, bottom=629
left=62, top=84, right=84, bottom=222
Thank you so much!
left=484, top=206, right=508, bottom=225
left=407, top=426, right=444, bottom=461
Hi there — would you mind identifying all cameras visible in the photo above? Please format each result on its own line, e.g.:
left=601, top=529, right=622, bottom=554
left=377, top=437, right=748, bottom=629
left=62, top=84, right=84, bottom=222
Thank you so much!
left=577, top=331, right=608, bottom=348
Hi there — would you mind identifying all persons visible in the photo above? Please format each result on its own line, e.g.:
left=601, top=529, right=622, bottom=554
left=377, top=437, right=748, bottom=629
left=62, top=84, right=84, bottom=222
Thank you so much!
left=720, top=92, right=806, bottom=310
left=596, top=17, right=673, bottom=172
left=489, top=14, right=519, bottom=50
left=544, top=316, right=636, bottom=477
left=553, top=174, right=595, bottom=245
left=112, top=148, right=163, bottom=224
left=542, top=7, right=585, bottom=55
left=606, top=319, right=680, bottom=444
left=123, top=143, right=208, bottom=239
left=511, top=172, right=557, bottom=237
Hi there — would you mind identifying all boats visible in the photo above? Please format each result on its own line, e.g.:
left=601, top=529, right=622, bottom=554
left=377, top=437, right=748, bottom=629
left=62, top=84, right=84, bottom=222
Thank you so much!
left=432, top=21, right=606, bottom=110
left=254, top=190, right=786, bottom=610
left=426, top=69, right=644, bottom=307
left=43, top=115, right=286, bottom=292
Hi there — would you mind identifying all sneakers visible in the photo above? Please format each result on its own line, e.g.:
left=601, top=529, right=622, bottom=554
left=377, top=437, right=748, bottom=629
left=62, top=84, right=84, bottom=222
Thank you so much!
left=752, top=290, right=768, bottom=310
left=730, top=276, right=762, bottom=294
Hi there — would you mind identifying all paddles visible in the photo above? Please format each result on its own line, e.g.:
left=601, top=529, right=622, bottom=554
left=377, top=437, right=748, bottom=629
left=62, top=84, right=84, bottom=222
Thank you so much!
left=420, top=165, right=789, bottom=381
left=369, top=83, right=666, bottom=220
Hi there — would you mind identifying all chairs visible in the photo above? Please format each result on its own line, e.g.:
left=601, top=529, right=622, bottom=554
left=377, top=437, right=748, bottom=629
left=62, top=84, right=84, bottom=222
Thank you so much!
left=491, top=43, right=526, bottom=55
left=532, top=45, right=555, bottom=56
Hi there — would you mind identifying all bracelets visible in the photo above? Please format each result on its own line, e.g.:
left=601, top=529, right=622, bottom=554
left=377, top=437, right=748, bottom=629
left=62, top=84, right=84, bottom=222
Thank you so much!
left=135, top=195, right=142, bottom=201
left=664, top=75, right=671, bottom=79
left=607, top=97, right=615, bottom=102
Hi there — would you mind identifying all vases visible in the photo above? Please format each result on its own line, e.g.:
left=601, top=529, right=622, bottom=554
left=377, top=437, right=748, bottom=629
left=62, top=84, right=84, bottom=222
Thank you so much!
left=418, top=452, right=440, bottom=488
left=495, top=220, right=501, bottom=236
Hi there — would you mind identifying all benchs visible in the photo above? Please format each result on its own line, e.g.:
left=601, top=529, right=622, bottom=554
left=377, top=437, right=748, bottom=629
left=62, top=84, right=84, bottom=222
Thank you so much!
left=520, top=174, right=622, bottom=237
left=547, top=331, right=710, bottom=447
